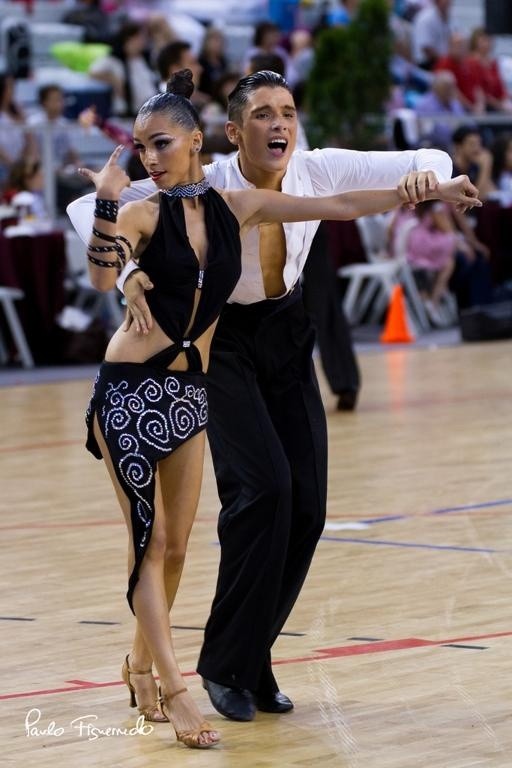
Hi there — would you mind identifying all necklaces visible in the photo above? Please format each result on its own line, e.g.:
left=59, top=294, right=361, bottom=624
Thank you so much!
left=158, top=176, right=212, bottom=199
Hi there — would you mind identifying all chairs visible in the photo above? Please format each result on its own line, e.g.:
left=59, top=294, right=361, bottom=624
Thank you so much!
left=0, top=0, right=512, bottom=366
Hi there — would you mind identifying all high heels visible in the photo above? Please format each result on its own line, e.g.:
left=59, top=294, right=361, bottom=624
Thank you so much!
left=122, top=654, right=170, bottom=721
left=156, top=685, right=220, bottom=748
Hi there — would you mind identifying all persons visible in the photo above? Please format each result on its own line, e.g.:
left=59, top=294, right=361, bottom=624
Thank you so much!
left=1, top=0, right=512, bottom=329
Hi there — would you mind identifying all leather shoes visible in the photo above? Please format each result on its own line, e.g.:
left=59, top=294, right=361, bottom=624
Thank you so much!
left=203, top=677, right=293, bottom=721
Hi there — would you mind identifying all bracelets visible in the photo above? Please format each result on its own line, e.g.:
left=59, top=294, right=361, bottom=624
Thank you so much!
left=84, top=195, right=120, bottom=270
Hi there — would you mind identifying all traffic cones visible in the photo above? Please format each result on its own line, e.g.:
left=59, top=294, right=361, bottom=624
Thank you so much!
left=378, top=286, right=416, bottom=346
